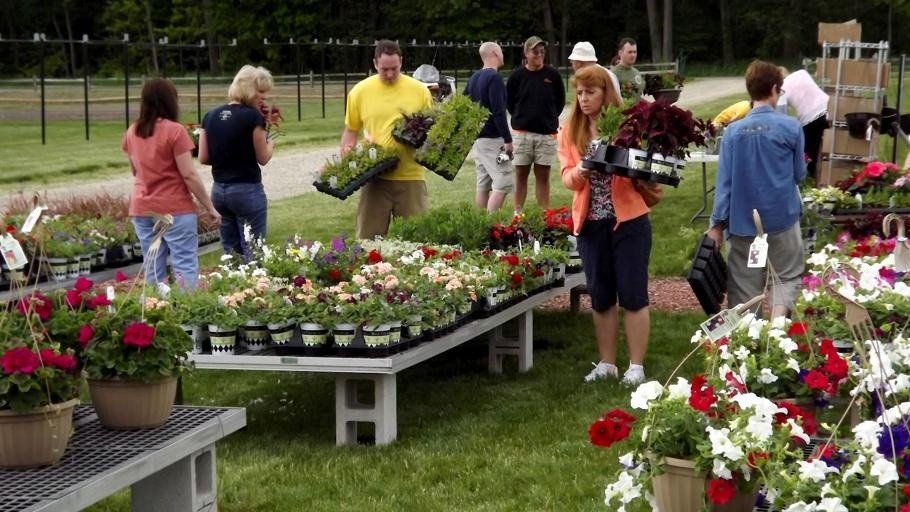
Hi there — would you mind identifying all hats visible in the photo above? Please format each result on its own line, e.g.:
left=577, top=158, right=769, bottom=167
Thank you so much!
left=568, top=40, right=599, bottom=62
left=524, top=35, right=548, bottom=50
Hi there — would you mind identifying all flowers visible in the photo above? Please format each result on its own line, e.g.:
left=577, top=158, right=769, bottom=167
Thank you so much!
left=73, top=271, right=195, bottom=384
left=0, top=286, right=82, bottom=412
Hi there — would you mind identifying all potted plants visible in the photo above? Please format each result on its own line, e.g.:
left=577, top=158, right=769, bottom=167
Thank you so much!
left=595, top=93, right=717, bottom=179
left=0, top=186, right=220, bottom=282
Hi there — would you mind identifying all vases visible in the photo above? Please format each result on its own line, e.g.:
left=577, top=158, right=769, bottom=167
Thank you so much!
left=85, top=377, right=177, bottom=430
left=0, top=398, right=81, bottom=469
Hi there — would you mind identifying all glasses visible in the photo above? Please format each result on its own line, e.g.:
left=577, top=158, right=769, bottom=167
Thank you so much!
left=779, top=88, right=786, bottom=98
left=530, top=49, right=545, bottom=55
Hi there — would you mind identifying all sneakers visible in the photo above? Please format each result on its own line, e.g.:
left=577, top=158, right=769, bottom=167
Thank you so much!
left=618, top=359, right=646, bottom=388
left=584, top=360, right=619, bottom=384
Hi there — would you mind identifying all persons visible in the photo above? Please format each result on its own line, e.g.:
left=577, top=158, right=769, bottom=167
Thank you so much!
left=609, top=36, right=647, bottom=104
left=555, top=65, right=662, bottom=388
left=462, top=41, right=518, bottom=215
left=122, top=79, right=224, bottom=295
left=341, top=41, right=437, bottom=239
left=504, top=36, right=567, bottom=215
left=706, top=60, right=807, bottom=325
left=776, top=64, right=831, bottom=179
left=713, top=100, right=755, bottom=131
left=198, top=63, right=278, bottom=271
left=568, top=40, right=623, bottom=104
